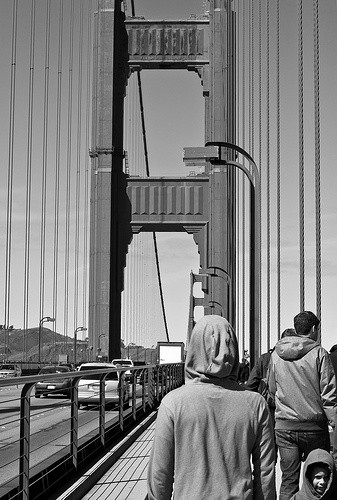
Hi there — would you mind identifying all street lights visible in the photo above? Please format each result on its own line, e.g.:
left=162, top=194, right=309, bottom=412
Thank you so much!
left=209, top=301, right=225, bottom=317
left=98, top=333, right=105, bottom=355
left=73, top=326, right=87, bottom=363
left=39, top=317, right=55, bottom=362
left=199, top=266, right=234, bottom=325
left=179, top=139, right=260, bottom=377
left=128, top=342, right=155, bottom=365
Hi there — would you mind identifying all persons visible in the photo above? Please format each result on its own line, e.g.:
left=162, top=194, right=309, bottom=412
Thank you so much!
left=266, top=310, right=337, bottom=500
left=145, top=315, right=278, bottom=500
left=235, top=357, right=250, bottom=385
left=247, top=328, right=297, bottom=411
left=328, top=344, right=337, bottom=369
left=287, top=449, right=337, bottom=500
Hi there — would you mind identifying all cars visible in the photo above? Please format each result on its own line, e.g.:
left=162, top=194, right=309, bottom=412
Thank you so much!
left=35, top=359, right=177, bottom=409
left=0, top=364, right=21, bottom=378
